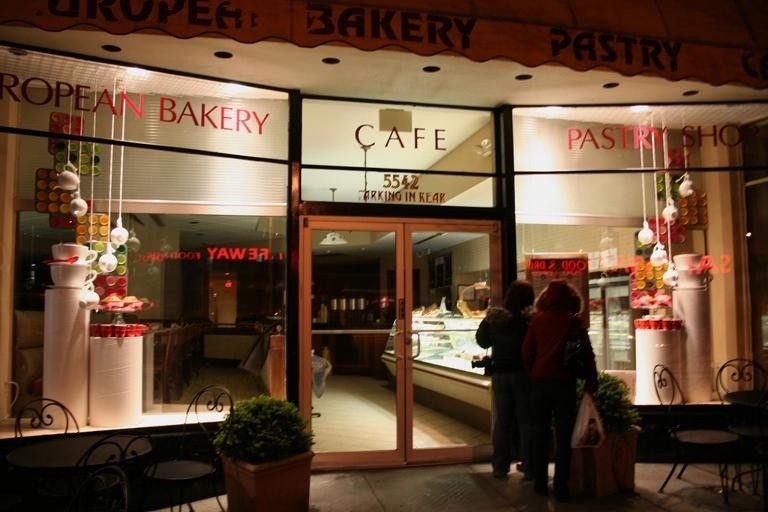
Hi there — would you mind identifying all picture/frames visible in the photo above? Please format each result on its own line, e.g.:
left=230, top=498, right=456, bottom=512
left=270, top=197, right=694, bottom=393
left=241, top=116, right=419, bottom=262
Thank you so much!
left=458, top=283, right=475, bottom=302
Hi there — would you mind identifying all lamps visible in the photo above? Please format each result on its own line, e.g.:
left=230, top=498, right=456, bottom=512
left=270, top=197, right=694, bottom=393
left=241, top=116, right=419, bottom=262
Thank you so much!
left=319, top=188, right=348, bottom=244
left=58, top=78, right=130, bottom=309
left=638, top=108, right=694, bottom=286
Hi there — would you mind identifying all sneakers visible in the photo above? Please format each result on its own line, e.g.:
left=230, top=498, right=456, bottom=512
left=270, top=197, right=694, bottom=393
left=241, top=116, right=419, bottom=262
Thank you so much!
left=492, top=457, right=569, bottom=502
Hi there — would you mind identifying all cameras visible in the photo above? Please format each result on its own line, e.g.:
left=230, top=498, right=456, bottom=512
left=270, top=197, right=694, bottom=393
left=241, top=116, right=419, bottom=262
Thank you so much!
left=472, top=356, right=493, bottom=376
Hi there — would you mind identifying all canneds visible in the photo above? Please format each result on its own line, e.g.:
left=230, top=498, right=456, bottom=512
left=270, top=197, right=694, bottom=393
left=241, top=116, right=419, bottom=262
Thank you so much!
left=330, top=298, right=366, bottom=311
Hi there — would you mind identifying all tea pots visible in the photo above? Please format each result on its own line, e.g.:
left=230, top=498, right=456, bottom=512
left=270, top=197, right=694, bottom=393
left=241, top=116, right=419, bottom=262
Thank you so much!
left=639, top=178, right=695, bottom=285
left=55, top=163, right=89, bottom=217
left=98, top=217, right=129, bottom=273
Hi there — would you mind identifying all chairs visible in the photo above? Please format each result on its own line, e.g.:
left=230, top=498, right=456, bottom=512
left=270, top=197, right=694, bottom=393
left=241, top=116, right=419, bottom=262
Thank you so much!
left=56, top=433, right=157, bottom=512
left=652, top=364, right=743, bottom=511
left=714, top=358, right=768, bottom=502
left=14, top=399, right=78, bottom=444
left=154, top=322, right=217, bottom=402
left=142, top=385, right=234, bottom=512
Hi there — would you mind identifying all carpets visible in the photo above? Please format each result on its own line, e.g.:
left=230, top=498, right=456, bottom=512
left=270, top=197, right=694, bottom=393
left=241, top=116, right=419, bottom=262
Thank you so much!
left=361, top=463, right=518, bottom=512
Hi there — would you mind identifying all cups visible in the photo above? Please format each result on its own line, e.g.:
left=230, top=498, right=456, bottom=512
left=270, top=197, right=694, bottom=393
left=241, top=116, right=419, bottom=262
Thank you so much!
left=633, top=319, right=682, bottom=330
left=48, top=242, right=97, bottom=287
left=93, top=324, right=145, bottom=338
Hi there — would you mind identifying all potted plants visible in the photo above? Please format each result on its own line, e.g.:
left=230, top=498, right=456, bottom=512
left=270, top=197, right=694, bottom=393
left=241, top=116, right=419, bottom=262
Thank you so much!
left=561, top=373, right=642, bottom=506
left=215, top=397, right=315, bottom=512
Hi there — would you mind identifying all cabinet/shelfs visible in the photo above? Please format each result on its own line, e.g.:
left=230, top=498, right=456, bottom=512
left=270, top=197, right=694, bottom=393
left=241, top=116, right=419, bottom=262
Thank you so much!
left=389, top=319, right=493, bottom=376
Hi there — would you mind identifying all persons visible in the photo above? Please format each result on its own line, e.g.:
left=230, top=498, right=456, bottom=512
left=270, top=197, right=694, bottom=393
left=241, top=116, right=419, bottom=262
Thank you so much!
left=476, top=279, right=599, bottom=503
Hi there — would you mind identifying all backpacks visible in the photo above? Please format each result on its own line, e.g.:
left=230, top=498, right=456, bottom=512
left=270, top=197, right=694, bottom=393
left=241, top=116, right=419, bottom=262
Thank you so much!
left=558, top=328, right=595, bottom=375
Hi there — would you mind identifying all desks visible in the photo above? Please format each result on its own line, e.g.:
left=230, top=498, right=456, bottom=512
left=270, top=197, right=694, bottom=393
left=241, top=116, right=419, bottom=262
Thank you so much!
left=6, top=434, right=152, bottom=512
